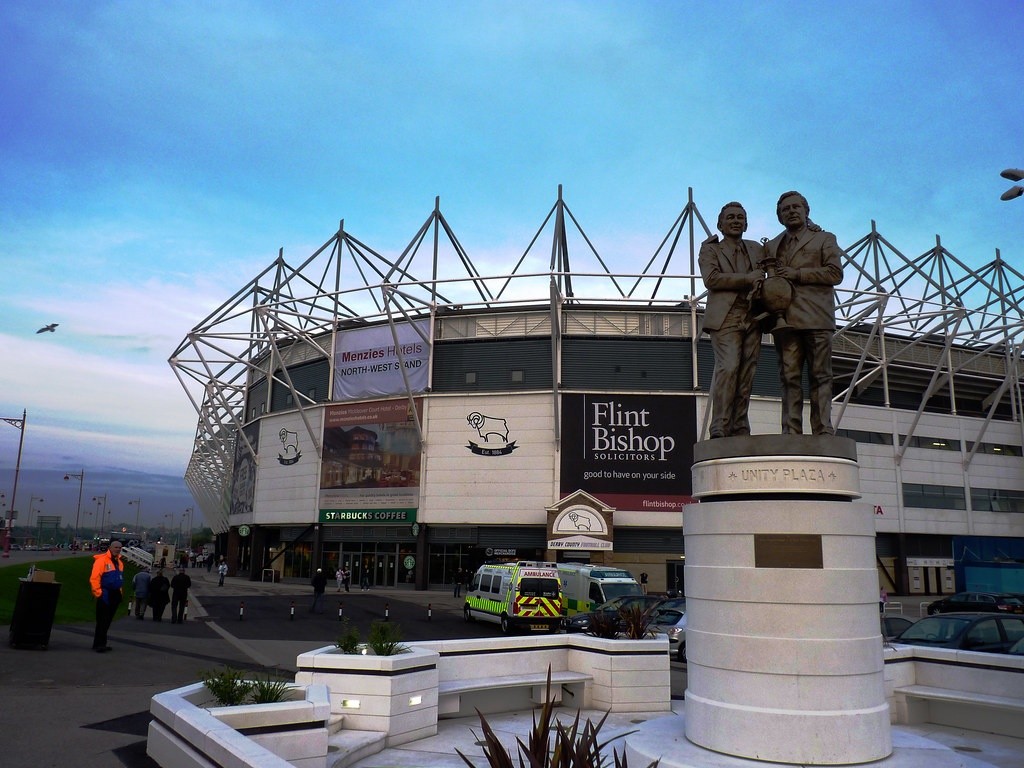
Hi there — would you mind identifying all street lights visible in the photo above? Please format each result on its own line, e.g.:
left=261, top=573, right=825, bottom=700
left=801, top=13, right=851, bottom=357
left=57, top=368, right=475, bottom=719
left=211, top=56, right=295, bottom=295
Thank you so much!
left=26, top=494, right=44, bottom=533
left=127, top=500, right=140, bottom=533
left=63, top=472, right=83, bottom=536
left=81, top=493, right=111, bottom=532
left=165, top=506, right=193, bottom=546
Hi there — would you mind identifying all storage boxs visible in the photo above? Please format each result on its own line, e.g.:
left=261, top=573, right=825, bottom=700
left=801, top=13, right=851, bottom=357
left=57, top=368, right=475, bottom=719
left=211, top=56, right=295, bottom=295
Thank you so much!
left=32, top=571, right=55, bottom=582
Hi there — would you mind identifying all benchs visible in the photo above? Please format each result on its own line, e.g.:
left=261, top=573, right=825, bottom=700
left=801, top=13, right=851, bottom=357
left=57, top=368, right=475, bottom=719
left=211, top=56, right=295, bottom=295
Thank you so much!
left=894, top=684, right=1024, bottom=725
left=438, top=669, right=592, bottom=714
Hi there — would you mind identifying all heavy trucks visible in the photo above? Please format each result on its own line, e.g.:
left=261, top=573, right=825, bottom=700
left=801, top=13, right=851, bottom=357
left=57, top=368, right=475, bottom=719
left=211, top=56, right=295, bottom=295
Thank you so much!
left=555, top=561, right=645, bottom=632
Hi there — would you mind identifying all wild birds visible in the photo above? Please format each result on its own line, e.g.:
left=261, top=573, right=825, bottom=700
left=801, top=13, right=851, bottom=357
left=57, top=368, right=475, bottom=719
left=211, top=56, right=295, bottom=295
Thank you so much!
left=36, top=323, right=58, bottom=333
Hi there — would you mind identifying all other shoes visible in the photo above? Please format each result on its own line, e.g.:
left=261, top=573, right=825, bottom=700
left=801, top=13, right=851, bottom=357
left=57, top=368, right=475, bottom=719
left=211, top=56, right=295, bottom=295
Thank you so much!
left=92, top=645, right=113, bottom=652
left=337, top=590, right=340, bottom=591
left=136, top=614, right=144, bottom=620
left=319, top=610, right=324, bottom=614
left=153, top=616, right=162, bottom=622
left=178, top=619, right=184, bottom=624
left=368, top=589, right=370, bottom=591
left=362, top=589, right=364, bottom=591
left=171, top=619, right=177, bottom=624
left=344, top=591, right=349, bottom=592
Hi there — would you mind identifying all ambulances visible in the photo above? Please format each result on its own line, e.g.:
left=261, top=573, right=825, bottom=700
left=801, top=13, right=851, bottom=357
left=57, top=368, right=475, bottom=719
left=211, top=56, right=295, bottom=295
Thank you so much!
left=464, top=560, right=566, bottom=635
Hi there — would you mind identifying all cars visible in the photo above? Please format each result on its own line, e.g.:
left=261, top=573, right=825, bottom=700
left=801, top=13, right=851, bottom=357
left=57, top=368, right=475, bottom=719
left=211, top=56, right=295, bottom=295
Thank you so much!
left=890, top=612, right=1024, bottom=658
left=9, top=540, right=109, bottom=552
left=880, top=613, right=939, bottom=639
left=560, top=595, right=662, bottom=634
left=926, top=591, right=1024, bottom=621
left=642, top=603, right=687, bottom=663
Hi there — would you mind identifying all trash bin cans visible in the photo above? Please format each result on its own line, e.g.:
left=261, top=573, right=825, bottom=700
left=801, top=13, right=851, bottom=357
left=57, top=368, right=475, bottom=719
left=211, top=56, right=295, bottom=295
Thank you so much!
left=9, top=576, right=63, bottom=650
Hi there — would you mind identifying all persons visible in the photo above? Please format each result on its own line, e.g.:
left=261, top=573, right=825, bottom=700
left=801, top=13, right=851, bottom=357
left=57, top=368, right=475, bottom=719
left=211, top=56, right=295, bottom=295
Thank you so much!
left=640, top=572, right=648, bottom=594
left=452, top=567, right=474, bottom=599
left=218, top=562, right=228, bottom=587
left=697, top=189, right=843, bottom=440
left=132, top=567, right=152, bottom=619
left=150, top=570, right=170, bottom=621
left=178, top=552, right=224, bottom=573
left=170, top=568, right=192, bottom=623
left=88, top=541, right=125, bottom=653
left=879, top=586, right=888, bottom=613
left=310, top=568, right=327, bottom=614
left=361, top=564, right=371, bottom=591
left=158, top=556, right=166, bottom=570
left=335, top=566, right=351, bottom=592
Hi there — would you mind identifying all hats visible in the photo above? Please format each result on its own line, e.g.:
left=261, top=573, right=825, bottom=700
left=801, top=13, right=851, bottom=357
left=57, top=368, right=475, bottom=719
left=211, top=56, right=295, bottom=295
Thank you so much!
left=317, top=568, right=322, bottom=573
